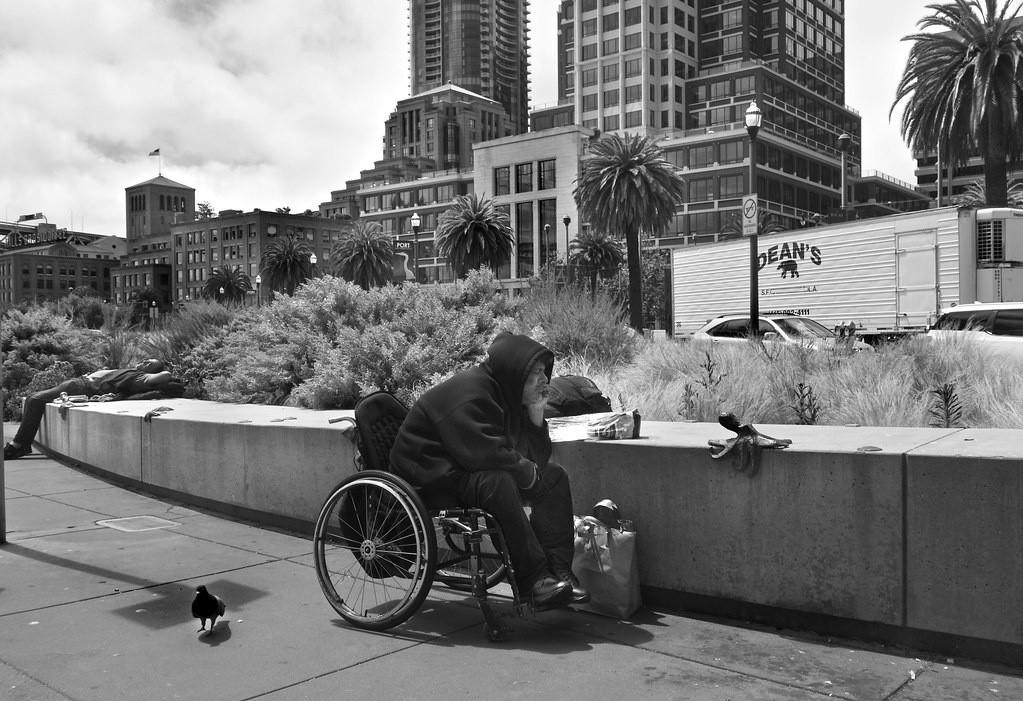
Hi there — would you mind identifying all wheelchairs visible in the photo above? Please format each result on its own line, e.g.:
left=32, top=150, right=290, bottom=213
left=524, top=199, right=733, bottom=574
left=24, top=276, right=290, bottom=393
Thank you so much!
left=313, top=392, right=570, bottom=645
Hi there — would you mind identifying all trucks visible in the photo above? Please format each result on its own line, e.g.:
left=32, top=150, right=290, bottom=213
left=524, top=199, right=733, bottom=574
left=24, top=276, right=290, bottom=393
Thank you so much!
left=672, top=203, right=1022, bottom=344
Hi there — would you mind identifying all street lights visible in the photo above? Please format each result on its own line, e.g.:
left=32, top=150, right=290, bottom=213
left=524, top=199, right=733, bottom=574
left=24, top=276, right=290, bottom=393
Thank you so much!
left=255, top=275, right=261, bottom=309
left=69, top=287, right=74, bottom=299
left=745, top=101, right=763, bottom=339
left=412, top=212, right=420, bottom=284
left=152, top=301, right=156, bottom=330
left=813, top=213, right=820, bottom=228
left=563, top=213, right=571, bottom=266
left=836, top=133, right=851, bottom=221
left=219, top=287, right=224, bottom=304
left=544, top=224, right=551, bottom=286
left=311, top=253, right=317, bottom=280
left=799, top=216, right=806, bottom=228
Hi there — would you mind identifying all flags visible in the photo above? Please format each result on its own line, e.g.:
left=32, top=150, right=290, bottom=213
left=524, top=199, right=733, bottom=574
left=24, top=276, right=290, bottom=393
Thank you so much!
left=150, top=148, right=159, bottom=156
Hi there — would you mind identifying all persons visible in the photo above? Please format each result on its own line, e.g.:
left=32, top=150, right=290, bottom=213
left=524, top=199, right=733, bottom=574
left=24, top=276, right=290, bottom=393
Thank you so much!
left=388, top=330, right=591, bottom=618
left=3, top=359, right=172, bottom=460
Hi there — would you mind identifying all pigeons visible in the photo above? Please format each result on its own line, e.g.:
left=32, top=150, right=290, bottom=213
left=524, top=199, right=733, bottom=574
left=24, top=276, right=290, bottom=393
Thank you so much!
left=192, top=584, right=226, bottom=636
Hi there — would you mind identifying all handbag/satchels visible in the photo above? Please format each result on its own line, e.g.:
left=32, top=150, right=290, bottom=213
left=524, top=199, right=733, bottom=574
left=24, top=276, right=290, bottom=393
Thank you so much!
left=570, top=515, right=642, bottom=621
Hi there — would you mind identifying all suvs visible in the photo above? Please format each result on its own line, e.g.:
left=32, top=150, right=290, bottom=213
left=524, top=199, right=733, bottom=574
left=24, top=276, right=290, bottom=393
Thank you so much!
left=689, top=311, right=877, bottom=354
left=922, top=302, right=1022, bottom=348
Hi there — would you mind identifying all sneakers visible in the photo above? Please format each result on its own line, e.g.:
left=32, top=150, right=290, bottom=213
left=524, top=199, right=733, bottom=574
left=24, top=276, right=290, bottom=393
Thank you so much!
left=554, top=569, right=591, bottom=604
left=519, top=574, right=573, bottom=606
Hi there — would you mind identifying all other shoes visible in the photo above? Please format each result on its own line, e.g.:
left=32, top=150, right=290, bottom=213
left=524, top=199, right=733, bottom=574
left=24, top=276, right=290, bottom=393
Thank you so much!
left=3, top=442, right=32, bottom=460
